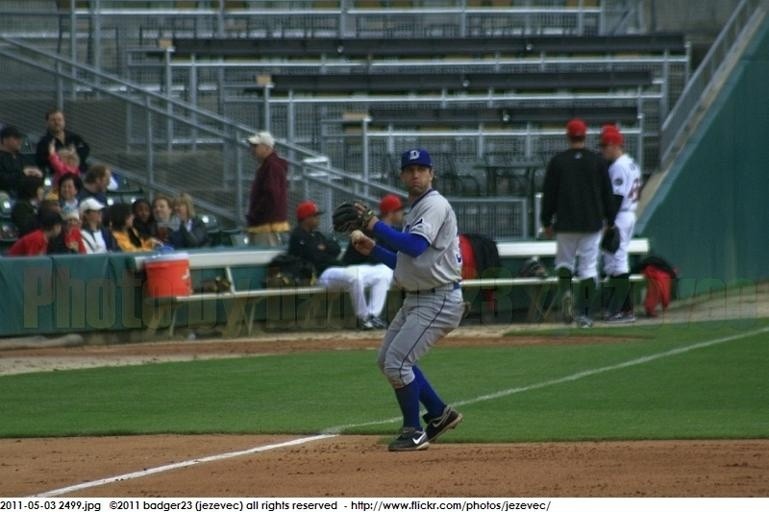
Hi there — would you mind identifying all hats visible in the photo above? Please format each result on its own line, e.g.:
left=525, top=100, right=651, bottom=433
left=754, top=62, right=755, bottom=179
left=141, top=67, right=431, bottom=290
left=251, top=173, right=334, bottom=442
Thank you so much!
left=398, top=148, right=433, bottom=170
left=378, top=195, right=404, bottom=214
left=246, top=131, right=278, bottom=148
left=294, top=201, right=326, bottom=220
left=565, top=117, right=627, bottom=148
left=60, top=197, right=105, bottom=221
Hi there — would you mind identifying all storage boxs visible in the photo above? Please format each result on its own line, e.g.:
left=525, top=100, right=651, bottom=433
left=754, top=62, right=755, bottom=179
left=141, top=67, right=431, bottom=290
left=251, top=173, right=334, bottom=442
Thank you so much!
left=145, top=250, right=194, bottom=299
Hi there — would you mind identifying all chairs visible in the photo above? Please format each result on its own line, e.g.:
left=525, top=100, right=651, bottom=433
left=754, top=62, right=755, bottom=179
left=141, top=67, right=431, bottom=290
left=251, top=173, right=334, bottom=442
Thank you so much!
left=0, top=123, right=263, bottom=250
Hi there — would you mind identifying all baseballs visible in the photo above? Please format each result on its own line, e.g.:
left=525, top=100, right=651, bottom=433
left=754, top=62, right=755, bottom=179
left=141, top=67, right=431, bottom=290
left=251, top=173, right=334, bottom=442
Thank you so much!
left=352, top=230, right=364, bottom=243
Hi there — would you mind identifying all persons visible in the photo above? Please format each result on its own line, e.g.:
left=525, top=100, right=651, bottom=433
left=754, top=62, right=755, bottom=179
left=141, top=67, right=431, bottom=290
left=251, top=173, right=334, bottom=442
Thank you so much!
left=244, top=130, right=291, bottom=247
left=284, top=201, right=395, bottom=330
left=598, top=124, right=643, bottom=323
left=0, top=108, right=207, bottom=256
left=540, top=119, right=616, bottom=329
left=339, top=193, right=408, bottom=265
left=349, top=147, right=466, bottom=452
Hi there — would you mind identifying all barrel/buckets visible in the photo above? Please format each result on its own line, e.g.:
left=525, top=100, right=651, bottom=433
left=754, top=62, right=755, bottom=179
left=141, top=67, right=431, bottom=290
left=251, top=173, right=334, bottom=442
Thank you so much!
left=145, top=245, right=192, bottom=297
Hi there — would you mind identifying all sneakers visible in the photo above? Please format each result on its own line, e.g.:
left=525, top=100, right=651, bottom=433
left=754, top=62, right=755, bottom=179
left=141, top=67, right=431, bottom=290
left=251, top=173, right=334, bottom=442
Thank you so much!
left=578, top=314, right=592, bottom=329
left=601, top=307, right=640, bottom=324
left=355, top=313, right=388, bottom=330
left=387, top=426, right=430, bottom=451
left=560, top=290, right=573, bottom=323
left=422, top=406, right=464, bottom=442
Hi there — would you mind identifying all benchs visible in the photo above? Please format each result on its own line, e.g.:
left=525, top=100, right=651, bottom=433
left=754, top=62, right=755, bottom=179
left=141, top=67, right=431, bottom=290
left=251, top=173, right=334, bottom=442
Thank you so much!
left=131, top=237, right=666, bottom=343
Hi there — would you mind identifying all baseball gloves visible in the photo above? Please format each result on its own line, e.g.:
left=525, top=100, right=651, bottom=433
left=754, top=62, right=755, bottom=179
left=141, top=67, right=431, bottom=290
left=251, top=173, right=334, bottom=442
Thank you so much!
left=601, top=225, right=620, bottom=255
left=332, top=200, right=375, bottom=231
